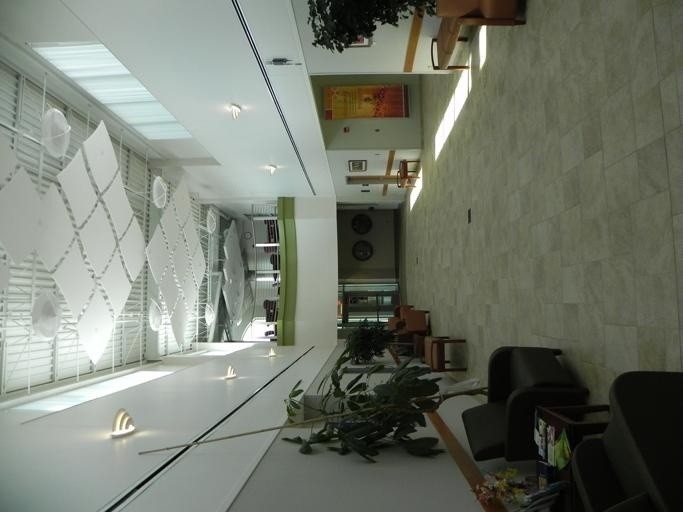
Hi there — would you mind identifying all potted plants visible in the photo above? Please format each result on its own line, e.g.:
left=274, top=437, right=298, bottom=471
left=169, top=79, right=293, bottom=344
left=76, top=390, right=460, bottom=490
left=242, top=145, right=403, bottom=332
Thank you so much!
left=346, top=318, right=425, bottom=364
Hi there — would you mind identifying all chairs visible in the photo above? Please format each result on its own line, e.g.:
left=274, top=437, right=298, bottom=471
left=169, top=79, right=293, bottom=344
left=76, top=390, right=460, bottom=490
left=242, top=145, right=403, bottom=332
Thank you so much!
left=387, top=1, right=681, bottom=509
left=263, top=220, right=280, bottom=342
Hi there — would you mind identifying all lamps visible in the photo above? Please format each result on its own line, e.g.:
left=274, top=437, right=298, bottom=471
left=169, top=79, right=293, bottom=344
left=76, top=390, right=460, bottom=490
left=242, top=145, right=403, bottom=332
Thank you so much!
left=33, top=102, right=275, bottom=439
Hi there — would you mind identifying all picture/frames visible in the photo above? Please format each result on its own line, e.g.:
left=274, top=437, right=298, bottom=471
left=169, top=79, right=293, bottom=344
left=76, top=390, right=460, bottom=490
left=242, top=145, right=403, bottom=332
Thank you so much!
left=348, top=159, right=374, bottom=262
